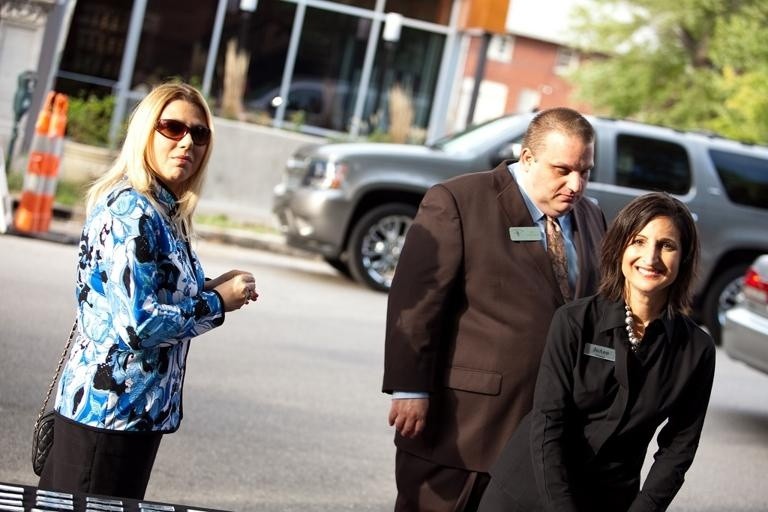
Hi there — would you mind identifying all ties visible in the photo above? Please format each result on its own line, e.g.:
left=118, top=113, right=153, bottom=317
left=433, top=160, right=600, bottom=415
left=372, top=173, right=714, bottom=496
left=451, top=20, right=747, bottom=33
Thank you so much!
left=546, top=215, right=574, bottom=305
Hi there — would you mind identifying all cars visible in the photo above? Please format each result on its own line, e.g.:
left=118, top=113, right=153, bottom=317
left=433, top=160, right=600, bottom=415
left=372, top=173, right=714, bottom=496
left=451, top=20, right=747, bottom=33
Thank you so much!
left=720, top=253, right=768, bottom=375
left=256, top=79, right=430, bottom=137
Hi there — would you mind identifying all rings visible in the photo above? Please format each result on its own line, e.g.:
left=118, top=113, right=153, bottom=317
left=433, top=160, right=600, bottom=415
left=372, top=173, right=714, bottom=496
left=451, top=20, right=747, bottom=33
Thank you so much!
left=244, top=291, right=251, bottom=302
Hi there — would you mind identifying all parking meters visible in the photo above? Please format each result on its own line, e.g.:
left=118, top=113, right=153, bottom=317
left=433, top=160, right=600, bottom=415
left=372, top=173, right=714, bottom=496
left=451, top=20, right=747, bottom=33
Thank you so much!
left=3, top=69, right=39, bottom=173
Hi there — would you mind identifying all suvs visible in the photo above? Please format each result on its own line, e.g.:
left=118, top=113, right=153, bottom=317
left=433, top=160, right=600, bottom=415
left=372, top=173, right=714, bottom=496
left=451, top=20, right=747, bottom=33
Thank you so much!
left=272, top=109, right=768, bottom=347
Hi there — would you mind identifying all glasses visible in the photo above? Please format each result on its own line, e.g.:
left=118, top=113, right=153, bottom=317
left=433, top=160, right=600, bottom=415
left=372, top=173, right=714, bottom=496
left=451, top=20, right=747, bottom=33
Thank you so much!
left=153, top=119, right=211, bottom=147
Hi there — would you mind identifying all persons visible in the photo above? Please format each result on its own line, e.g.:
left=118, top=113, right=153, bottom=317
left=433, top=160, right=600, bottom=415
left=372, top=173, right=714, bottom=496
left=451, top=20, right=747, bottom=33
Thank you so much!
left=379, top=105, right=612, bottom=512
left=33, top=80, right=260, bottom=503
left=475, top=188, right=716, bottom=512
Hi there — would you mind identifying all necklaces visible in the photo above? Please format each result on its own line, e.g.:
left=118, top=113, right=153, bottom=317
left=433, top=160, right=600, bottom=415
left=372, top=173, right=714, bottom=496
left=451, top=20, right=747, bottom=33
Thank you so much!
left=621, top=297, right=641, bottom=353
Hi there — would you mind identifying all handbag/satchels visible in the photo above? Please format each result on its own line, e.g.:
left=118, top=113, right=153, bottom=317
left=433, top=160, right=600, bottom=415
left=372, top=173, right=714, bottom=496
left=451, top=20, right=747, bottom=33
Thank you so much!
left=32, top=407, right=71, bottom=477
